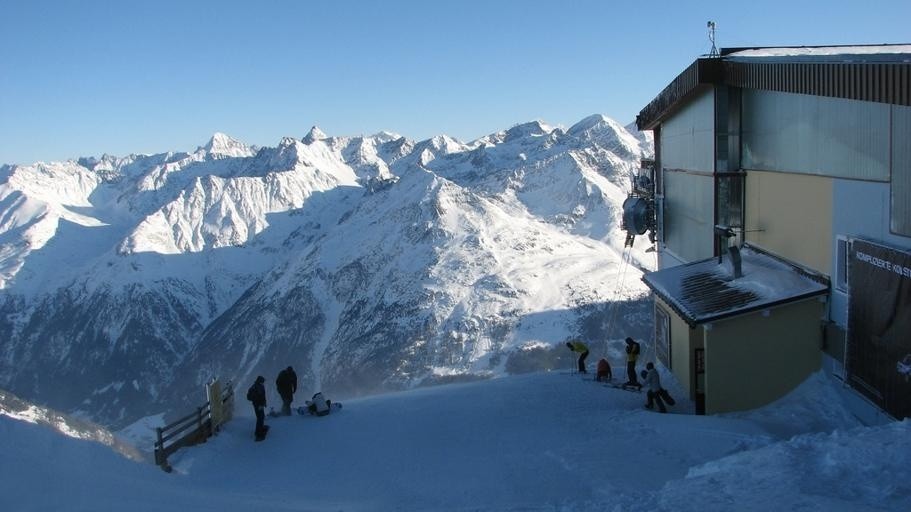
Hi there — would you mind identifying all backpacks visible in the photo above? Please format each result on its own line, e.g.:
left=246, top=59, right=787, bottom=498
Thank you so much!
left=247, top=382, right=258, bottom=403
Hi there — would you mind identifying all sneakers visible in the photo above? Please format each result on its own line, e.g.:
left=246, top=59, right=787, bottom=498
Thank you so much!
left=644, top=404, right=653, bottom=409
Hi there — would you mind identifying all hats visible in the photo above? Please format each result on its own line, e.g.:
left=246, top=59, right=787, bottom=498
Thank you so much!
left=647, top=363, right=653, bottom=369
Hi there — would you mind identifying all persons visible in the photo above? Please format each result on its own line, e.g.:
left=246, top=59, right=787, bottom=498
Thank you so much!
left=245, top=375, right=267, bottom=442
left=638, top=362, right=667, bottom=413
left=565, top=341, right=589, bottom=374
left=304, top=391, right=330, bottom=417
left=596, top=357, right=612, bottom=384
left=623, top=336, right=640, bottom=386
left=274, top=366, right=297, bottom=416
